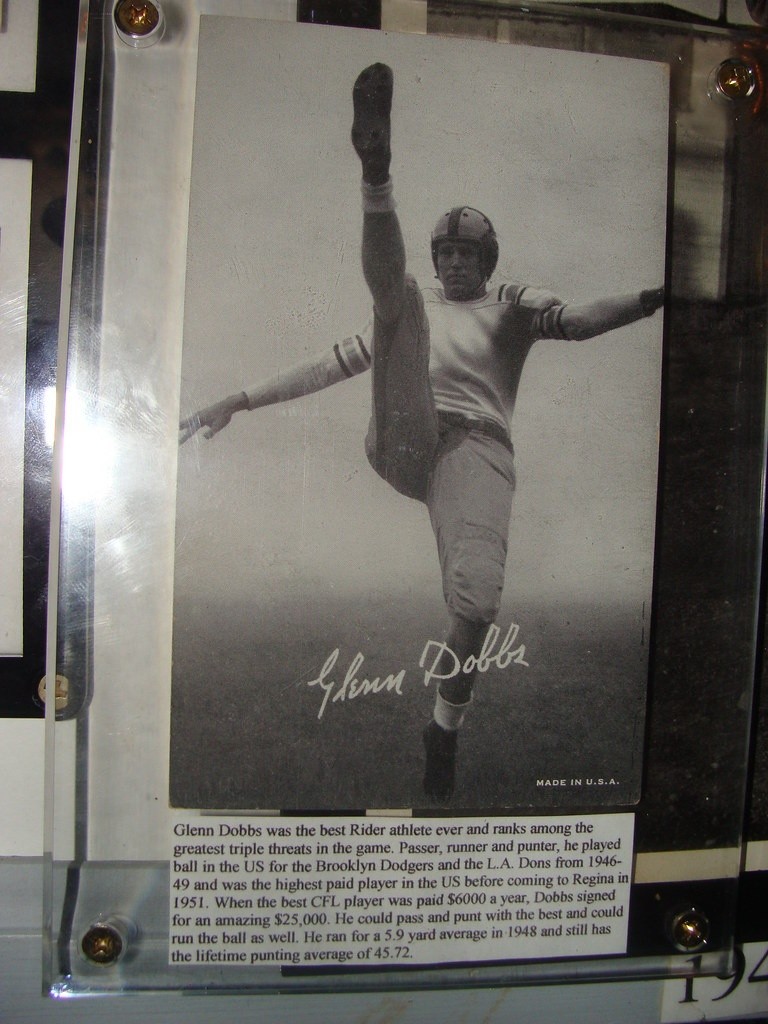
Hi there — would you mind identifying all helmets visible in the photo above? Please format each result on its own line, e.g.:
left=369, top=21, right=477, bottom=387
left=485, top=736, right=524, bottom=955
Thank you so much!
left=430, top=205, right=500, bottom=277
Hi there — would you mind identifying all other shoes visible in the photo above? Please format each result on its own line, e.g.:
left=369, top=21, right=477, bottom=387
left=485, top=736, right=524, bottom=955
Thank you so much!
left=419, top=723, right=458, bottom=806
left=348, top=63, right=394, bottom=166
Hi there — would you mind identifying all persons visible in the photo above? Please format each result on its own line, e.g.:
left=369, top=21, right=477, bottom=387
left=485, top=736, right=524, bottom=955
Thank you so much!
left=178, top=63, right=664, bottom=800
